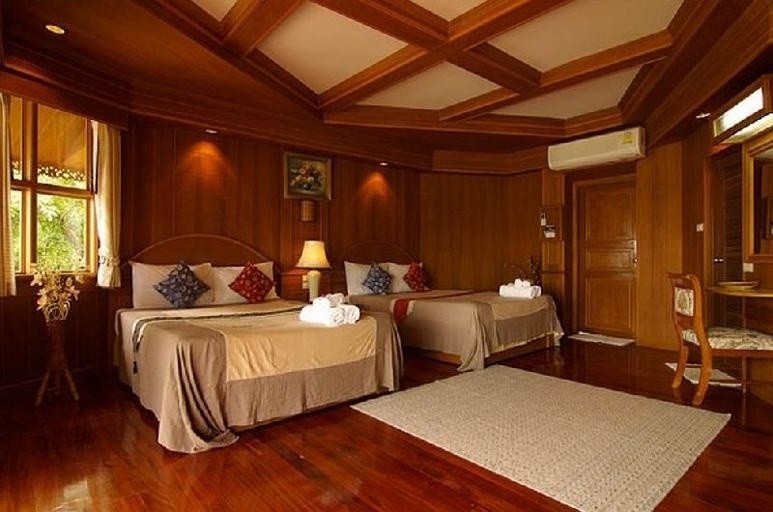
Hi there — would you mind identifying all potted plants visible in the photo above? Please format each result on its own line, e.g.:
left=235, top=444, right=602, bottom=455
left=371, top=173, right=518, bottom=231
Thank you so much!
left=26, top=261, right=84, bottom=409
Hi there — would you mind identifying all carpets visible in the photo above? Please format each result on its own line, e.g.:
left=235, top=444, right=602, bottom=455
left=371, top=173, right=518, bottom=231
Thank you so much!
left=349, top=363, right=731, bottom=512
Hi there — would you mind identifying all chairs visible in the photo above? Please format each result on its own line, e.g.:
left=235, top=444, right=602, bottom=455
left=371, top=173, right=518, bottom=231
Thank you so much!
left=666, top=268, right=773, bottom=406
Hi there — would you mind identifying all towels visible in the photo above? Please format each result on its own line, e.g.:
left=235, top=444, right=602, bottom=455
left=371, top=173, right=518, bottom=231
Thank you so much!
left=499, top=278, right=542, bottom=300
left=299, top=292, right=361, bottom=329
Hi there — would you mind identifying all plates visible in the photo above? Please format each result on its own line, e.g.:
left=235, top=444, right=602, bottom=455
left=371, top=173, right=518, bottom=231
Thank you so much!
left=717, top=281, right=760, bottom=292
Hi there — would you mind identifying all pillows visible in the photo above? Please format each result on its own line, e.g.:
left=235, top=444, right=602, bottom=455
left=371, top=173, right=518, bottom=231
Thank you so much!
left=127, top=256, right=280, bottom=311
left=344, top=260, right=434, bottom=297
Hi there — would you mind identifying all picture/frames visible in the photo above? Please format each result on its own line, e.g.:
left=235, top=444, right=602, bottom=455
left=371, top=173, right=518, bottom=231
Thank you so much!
left=281, top=152, right=334, bottom=202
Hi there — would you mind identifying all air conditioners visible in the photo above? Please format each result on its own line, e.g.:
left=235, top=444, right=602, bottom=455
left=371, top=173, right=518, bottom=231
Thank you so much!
left=547, top=125, right=646, bottom=172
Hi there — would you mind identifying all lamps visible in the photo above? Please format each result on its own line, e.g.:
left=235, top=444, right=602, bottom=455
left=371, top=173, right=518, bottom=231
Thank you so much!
left=292, top=231, right=335, bottom=301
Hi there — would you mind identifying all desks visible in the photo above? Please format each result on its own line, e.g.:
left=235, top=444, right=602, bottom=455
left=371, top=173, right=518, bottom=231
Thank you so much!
left=707, top=276, right=772, bottom=395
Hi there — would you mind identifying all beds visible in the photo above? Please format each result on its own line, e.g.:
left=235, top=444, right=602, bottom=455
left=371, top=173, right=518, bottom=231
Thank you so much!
left=107, top=233, right=405, bottom=456
left=332, top=238, right=565, bottom=372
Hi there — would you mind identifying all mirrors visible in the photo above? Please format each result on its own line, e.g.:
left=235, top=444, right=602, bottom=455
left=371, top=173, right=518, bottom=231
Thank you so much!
left=741, top=130, right=772, bottom=265
